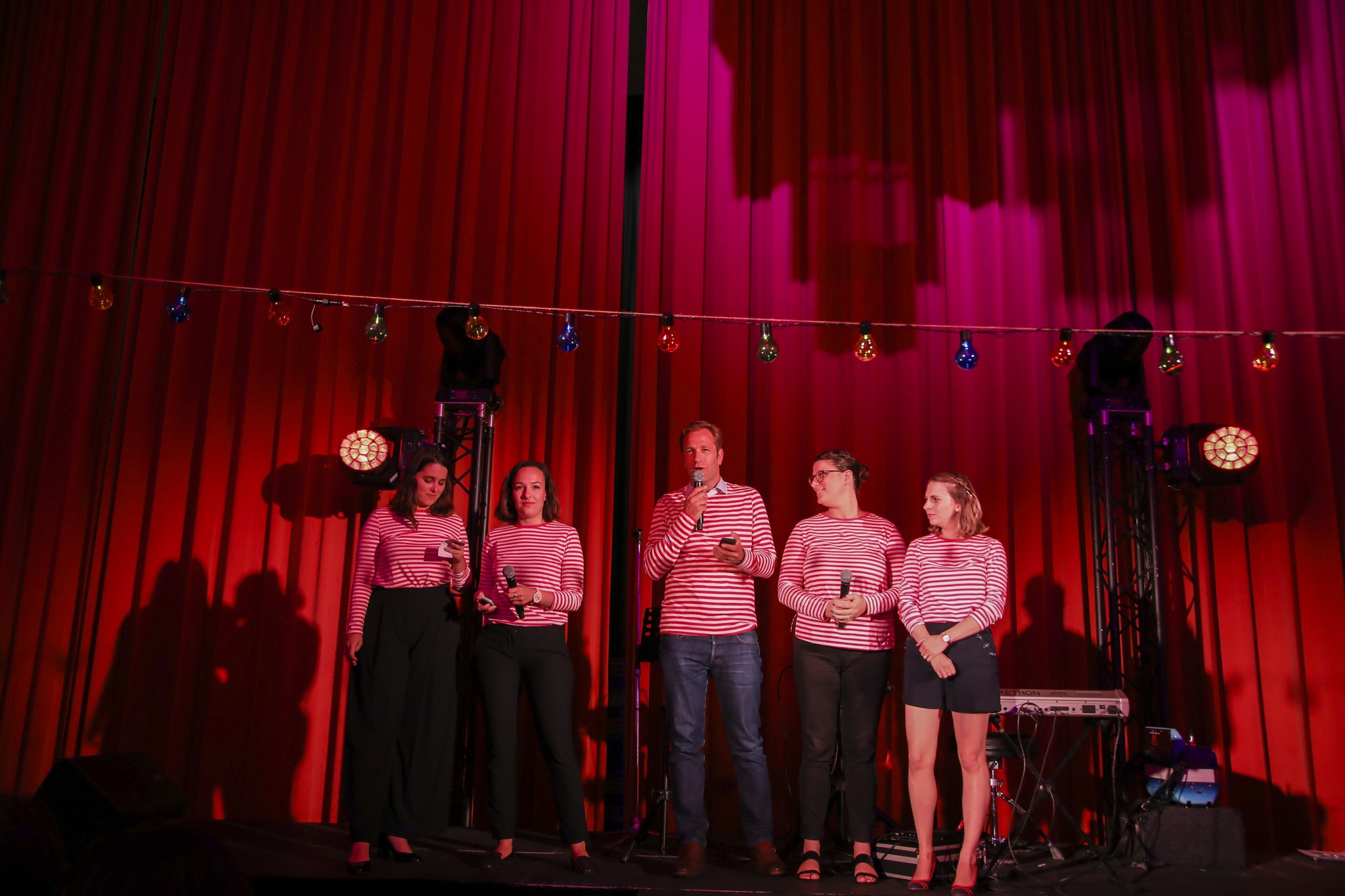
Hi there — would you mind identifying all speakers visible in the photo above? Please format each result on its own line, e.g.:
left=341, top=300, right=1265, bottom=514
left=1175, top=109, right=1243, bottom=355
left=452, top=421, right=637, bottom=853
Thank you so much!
left=35, top=749, right=190, bottom=864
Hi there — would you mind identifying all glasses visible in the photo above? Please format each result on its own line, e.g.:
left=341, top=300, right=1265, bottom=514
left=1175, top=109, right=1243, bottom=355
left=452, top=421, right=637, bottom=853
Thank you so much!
left=808, top=470, right=846, bottom=485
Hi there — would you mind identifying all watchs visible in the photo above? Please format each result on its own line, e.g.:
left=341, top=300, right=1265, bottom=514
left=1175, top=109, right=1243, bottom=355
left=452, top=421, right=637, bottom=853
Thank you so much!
left=531, top=588, right=542, bottom=603
left=941, top=631, right=952, bottom=646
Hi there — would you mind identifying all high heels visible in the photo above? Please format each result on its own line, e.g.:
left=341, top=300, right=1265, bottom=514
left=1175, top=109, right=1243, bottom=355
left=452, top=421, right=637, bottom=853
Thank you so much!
left=347, top=850, right=372, bottom=874
left=379, top=832, right=422, bottom=863
left=908, top=855, right=940, bottom=891
left=950, top=862, right=980, bottom=896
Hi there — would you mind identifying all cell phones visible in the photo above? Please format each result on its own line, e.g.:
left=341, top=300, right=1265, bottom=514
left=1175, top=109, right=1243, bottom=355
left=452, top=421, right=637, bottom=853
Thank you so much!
left=718, top=538, right=736, bottom=548
left=480, top=595, right=495, bottom=606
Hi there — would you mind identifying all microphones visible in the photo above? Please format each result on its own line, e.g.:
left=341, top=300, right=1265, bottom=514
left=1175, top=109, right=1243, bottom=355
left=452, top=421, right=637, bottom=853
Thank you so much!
left=838, top=569, right=853, bottom=628
left=692, top=470, right=704, bottom=530
left=502, top=565, right=525, bottom=619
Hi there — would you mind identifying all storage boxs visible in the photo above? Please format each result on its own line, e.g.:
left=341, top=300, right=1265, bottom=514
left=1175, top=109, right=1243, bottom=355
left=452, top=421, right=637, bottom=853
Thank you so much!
left=1112, top=801, right=1246, bottom=868
left=872, top=841, right=987, bottom=882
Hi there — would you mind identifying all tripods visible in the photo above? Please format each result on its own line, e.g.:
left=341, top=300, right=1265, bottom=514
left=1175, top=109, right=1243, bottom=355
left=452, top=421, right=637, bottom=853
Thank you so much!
left=776, top=704, right=902, bottom=874
left=601, top=706, right=728, bottom=865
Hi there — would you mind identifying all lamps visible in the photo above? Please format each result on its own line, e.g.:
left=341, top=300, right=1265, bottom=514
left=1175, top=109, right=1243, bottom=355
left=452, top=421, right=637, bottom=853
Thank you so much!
left=1161, top=422, right=1261, bottom=491
left=337, top=424, right=427, bottom=491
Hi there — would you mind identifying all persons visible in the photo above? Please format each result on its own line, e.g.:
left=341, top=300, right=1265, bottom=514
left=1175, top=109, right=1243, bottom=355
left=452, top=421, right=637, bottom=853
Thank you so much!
left=643, top=420, right=788, bottom=881
left=776, top=447, right=908, bottom=887
left=894, top=470, right=1007, bottom=896
left=345, top=443, right=470, bottom=875
left=472, top=459, right=602, bottom=881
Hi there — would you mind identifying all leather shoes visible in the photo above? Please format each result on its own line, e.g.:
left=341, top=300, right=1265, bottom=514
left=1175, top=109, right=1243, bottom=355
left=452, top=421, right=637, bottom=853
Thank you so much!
left=749, top=840, right=786, bottom=876
left=479, top=844, right=518, bottom=871
left=674, top=842, right=711, bottom=879
left=570, top=853, right=598, bottom=874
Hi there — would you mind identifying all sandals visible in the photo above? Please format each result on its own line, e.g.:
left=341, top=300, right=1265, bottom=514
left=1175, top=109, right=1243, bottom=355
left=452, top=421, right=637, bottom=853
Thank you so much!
left=853, top=853, right=880, bottom=885
left=796, top=849, right=822, bottom=882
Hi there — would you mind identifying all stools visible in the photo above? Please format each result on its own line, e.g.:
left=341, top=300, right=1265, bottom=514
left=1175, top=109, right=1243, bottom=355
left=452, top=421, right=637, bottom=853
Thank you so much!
left=948, top=732, right=1037, bottom=867
